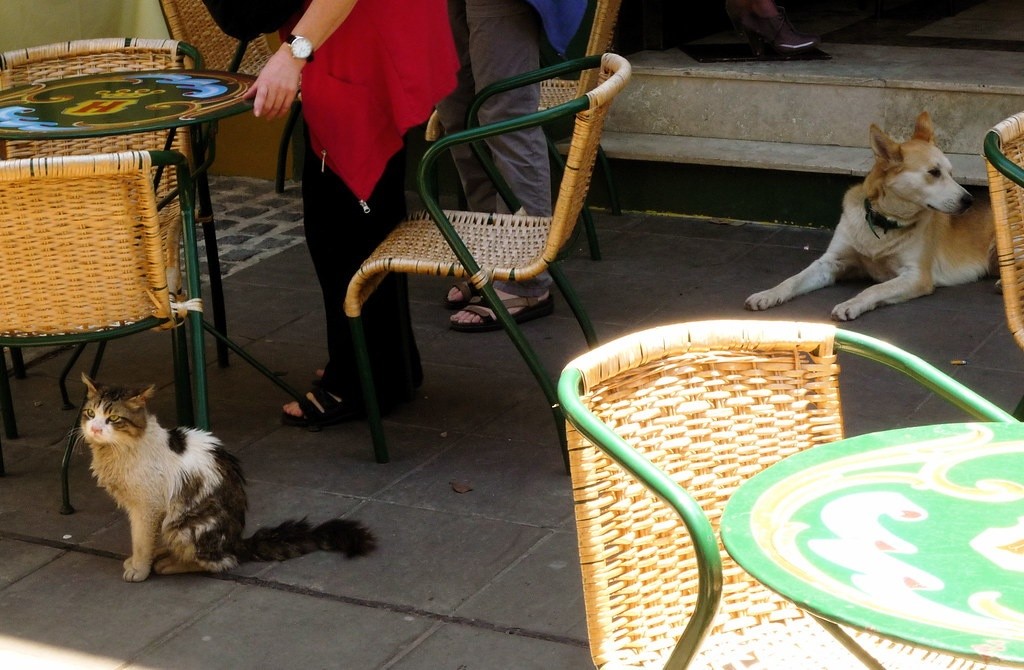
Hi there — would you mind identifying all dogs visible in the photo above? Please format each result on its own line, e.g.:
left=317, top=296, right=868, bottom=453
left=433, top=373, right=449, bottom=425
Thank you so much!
left=744, top=110, right=1024, bottom=321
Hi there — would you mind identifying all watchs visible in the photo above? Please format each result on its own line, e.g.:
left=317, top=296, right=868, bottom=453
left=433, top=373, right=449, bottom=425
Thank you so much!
left=284, top=33, right=314, bottom=63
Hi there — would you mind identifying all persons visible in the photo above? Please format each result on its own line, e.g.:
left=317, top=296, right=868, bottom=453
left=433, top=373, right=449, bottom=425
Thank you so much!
left=244, top=0, right=591, bottom=426
left=724, top=0, right=818, bottom=56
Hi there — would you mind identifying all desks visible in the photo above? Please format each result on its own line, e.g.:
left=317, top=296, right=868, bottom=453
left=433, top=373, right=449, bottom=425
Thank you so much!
left=0, top=69, right=304, bottom=514
left=718, top=423, right=1024, bottom=670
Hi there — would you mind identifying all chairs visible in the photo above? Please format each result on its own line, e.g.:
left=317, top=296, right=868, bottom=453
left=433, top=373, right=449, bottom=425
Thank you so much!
left=424, top=0, right=621, bottom=261
left=159, top=0, right=301, bottom=193
left=1, top=37, right=230, bottom=370
left=0, top=149, right=209, bottom=432
left=342, top=53, right=633, bottom=474
left=558, top=319, right=1024, bottom=670
left=983, top=111, right=1024, bottom=425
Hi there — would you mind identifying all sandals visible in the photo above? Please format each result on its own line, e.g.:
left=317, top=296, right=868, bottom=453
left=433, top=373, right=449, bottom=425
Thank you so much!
left=280, top=380, right=367, bottom=429
left=441, top=277, right=555, bottom=331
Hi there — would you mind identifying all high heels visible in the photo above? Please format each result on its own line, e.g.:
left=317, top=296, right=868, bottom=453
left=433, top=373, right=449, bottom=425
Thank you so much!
left=726, top=0, right=820, bottom=56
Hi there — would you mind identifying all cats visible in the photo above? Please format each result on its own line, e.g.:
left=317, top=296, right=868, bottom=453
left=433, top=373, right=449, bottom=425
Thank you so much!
left=73, top=371, right=379, bottom=583
